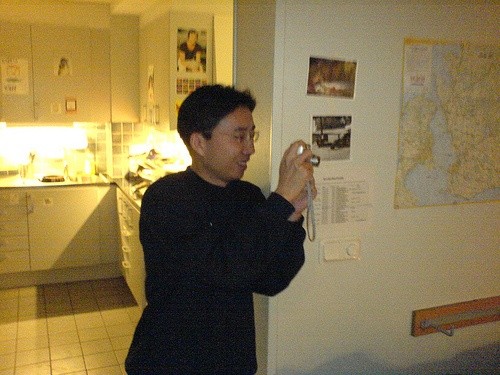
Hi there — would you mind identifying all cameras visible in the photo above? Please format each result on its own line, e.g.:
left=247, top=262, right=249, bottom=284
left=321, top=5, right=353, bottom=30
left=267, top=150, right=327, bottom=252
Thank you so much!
left=297, top=144, right=319, bottom=165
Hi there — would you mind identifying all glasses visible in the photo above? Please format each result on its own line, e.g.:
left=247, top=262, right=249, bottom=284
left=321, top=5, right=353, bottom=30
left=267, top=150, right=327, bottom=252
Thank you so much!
left=203, top=131, right=260, bottom=143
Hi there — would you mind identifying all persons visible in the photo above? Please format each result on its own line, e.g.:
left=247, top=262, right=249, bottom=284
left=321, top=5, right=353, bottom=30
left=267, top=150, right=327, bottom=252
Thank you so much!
left=125, top=84, right=317, bottom=375
left=178, top=30, right=202, bottom=72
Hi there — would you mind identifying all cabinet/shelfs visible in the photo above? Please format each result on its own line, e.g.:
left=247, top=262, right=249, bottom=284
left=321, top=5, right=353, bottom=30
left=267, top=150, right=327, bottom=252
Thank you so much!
left=116, top=185, right=146, bottom=311
left=0, top=20, right=111, bottom=122
left=139, top=9, right=215, bottom=133
left=0, top=183, right=116, bottom=291
